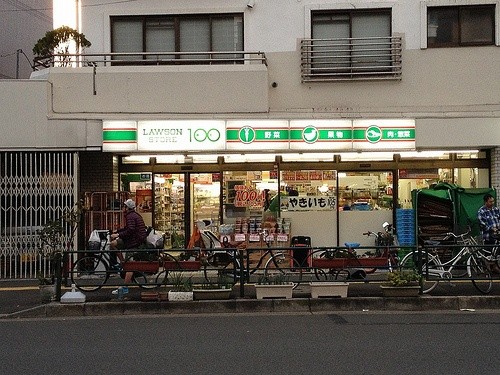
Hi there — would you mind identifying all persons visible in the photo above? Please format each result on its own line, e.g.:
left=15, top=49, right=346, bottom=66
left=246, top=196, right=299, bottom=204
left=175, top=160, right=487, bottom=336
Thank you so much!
left=478, top=194, right=500, bottom=273
left=110, top=198, right=148, bottom=294
left=267, top=190, right=298, bottom=216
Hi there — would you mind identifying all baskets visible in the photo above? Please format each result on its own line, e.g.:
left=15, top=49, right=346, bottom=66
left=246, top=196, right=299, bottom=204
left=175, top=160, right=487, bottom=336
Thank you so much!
left=377, top=235, right=394, bottom=245
left=86, top=240, right=101, bottom=253
left=468, top=235, right=484, bottom=252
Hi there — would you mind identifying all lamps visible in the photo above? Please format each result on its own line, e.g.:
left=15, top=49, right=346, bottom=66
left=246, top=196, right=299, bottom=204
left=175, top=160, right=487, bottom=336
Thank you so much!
left=247, top=0, right=256, bottom=8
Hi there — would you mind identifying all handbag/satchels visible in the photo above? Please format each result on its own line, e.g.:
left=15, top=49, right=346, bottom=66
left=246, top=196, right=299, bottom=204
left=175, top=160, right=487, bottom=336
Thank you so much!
left=88, top=230, right=101, bottom=242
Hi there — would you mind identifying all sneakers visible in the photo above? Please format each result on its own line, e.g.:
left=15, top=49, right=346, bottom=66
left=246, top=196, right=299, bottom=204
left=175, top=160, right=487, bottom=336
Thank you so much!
left=112, top=286, right=129, bottom=295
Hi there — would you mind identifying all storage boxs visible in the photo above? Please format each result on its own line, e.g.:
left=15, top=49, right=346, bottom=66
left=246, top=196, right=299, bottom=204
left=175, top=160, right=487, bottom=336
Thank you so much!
left=395, top=209, right=415, bottom=246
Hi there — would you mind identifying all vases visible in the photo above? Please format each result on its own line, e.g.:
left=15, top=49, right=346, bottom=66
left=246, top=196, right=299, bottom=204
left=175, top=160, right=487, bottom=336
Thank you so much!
left=309, top=281, right=349, bottom=298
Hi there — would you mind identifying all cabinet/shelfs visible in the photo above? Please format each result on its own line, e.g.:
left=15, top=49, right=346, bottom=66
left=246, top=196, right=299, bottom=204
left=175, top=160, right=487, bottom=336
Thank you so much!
left=221, top=219, right=292, bottom=268
left=223, top=180, right=264, bottom=220
left=154, top=184, right=222, bottom=231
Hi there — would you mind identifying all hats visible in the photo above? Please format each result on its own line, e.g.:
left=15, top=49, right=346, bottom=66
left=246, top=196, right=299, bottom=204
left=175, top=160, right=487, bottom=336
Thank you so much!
left=123, top=198, right=136, bottom=209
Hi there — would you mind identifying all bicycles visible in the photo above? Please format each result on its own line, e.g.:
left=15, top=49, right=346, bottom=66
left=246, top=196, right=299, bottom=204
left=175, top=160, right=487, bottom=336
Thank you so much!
left=313, top=224, right=404, bottom=282
left=399, top=224, right=493, bottom=294
left=70, top=229, right=169, bottom=290
left=203, top=230, right=303, bottom=290
left=441, top=217, right=500, bottom=278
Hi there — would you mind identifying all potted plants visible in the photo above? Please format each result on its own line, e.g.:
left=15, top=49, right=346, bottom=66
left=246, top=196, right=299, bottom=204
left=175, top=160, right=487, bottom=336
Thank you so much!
left=122, top=244, right=159, bottom=272
left=162, top=253, right=200, bottom=271
left=312, top=251, right=388, bottom=267
left=168, top=270, right=194, bottom=301
left=253, top=263, right=295, bottom=299
left=38, top=275, right=57, bottom=303
left=380, top=269, right=426, bottom=296
left=192, top=272, right=234, bottom=299
